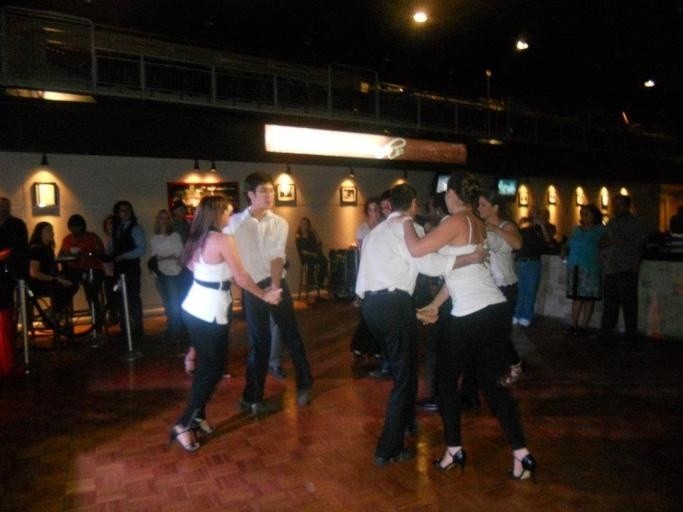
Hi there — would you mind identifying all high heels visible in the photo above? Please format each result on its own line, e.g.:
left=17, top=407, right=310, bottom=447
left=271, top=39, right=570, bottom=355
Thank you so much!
left=508, top=453, right=537, bottom=483
left=193, top=415, right=217, bottom=436
left=168, top=425, right=201, bottom=453
left=500, top=362, right=528, bottom=385
left=433, top=448, right=466, bottom=474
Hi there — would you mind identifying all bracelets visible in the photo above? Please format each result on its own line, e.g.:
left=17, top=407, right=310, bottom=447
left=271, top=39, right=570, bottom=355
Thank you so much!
left=401, top=215, right=413, bottom=222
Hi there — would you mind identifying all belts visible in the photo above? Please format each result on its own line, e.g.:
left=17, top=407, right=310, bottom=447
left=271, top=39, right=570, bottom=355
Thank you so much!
left=364, top=289, right=401, bottom=299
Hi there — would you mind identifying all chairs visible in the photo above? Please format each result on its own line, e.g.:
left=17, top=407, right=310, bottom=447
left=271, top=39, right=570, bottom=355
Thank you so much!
left=297, top=245, right=332, bottom=306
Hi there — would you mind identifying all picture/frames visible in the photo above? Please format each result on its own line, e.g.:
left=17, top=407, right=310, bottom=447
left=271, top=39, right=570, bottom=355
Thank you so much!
left=274, top=183, right=296, bottom=206
left=340, top=185, right=358, bottom=204
left=519, top=191, right=529, bottom=206
left=33, top=182, right=58, bottom=209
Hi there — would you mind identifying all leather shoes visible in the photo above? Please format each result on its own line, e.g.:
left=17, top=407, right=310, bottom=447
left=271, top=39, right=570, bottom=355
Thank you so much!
left=367, top=367, right=390, bottom=379
left=269, top=365, right=289, bottom=378
left=374, top=444, right=415, bottom=464
left=415, top=398, right=441, bottom=413
left=240, top=400, right=278, bottom=415
left=298, top=380, right=315, bottom=406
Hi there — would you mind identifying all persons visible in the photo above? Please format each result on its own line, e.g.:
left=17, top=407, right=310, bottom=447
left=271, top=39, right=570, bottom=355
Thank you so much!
left=559, top=204, right=625, bottom=335
left=475, top=190, right=528, bottom=386
left=389, top=173, right=539, bottom=484
left=295, top=217, right=328, bottom=304
left=104, top=200, right=146, bottom=337
left=168, top=199, right=191, bottom=248
left=355, top=184, right=491, bottom=466
left=222, top=170, right=313, bottom=416
left=26, top=220, right=79, bottom=322
left=57, top=213, right=106, bottom=318
left=149, top=208, right=183, bottom=328
left=166, top=194, right=282, bottom=452
left=539, top=208, right=557, bottom=255
left=101, top=215, right=119, bottom=325
left=511, top=206, right=549, bottom=328
left=414, top=193, right=481, bottom=415
left=349, top=196, right=382, bottom=359
left=592, top=195, right=646, bottom=339
left=0, top=194, right=29, bottom=318
left=369, top=189, right=425, bottom=379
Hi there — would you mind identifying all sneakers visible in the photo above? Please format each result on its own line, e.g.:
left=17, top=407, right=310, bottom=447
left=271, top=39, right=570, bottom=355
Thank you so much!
left=512, top=317, right=519, bottom=326
left=519, top=319, right=530, bottom=327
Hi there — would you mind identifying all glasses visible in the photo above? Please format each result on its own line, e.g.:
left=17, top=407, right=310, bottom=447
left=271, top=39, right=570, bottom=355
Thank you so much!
left=252, top=188, right=274, bottom=194
